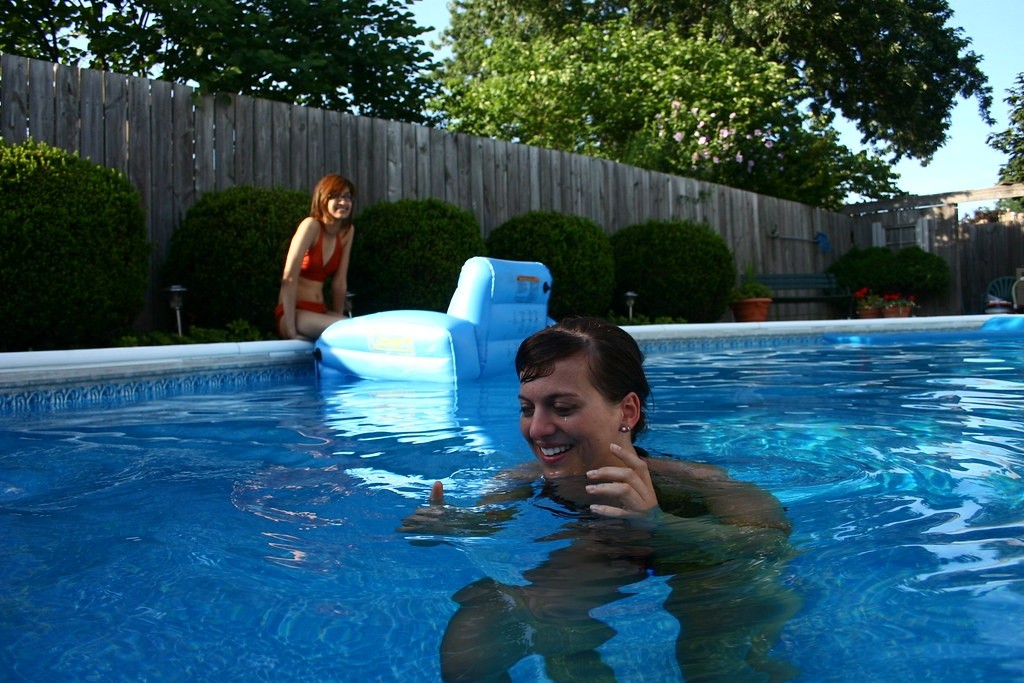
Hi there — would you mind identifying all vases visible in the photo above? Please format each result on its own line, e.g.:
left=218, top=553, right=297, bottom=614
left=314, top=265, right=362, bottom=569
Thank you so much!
left=881, top=307, right=900, bottom=318
left=899, top=306, right=912, bottom=317
left=856, top=307, right=882, bottom=318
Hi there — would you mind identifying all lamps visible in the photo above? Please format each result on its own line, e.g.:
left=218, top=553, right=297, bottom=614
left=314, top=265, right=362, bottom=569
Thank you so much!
left=625, top=290, right=637, bottom=320
left=168, top=283, right=185, bottom=336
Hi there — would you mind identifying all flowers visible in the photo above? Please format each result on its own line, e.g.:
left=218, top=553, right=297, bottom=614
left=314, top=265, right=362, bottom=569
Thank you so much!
left=880, top=293, right=903, bottom=307
left=855, top=287, right=883, bottom=307
left=900, top=295, right=921, bottom=308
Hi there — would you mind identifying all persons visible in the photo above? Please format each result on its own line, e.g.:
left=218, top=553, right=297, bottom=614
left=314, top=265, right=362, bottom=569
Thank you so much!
left=429, top=318, right=727, bottom=518
left=275, top=174, right=355, bottom=341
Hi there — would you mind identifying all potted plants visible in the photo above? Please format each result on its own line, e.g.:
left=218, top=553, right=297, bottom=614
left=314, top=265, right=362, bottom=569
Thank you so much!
left=728, top=261, right=774, bottom=322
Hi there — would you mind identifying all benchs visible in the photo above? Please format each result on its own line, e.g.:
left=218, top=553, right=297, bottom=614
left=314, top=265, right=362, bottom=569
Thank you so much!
left=739, top=272, right=854, bottom=319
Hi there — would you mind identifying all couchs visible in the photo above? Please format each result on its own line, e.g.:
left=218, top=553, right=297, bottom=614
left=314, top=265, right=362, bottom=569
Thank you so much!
left=312, top=256, right=557, bottom=382
